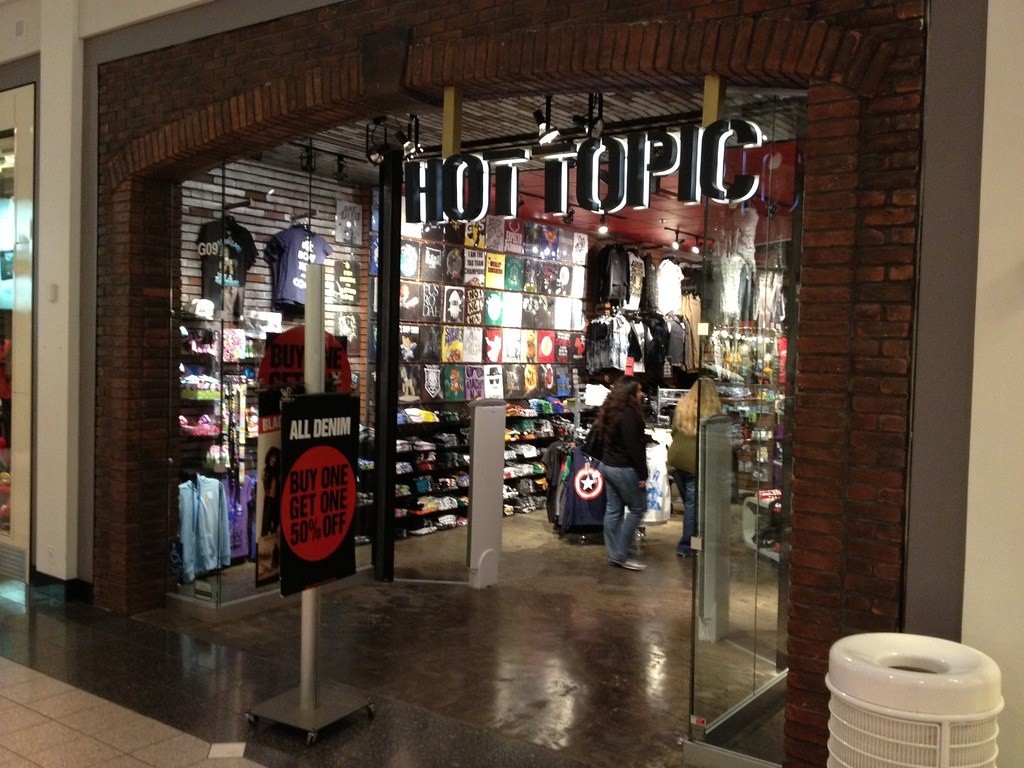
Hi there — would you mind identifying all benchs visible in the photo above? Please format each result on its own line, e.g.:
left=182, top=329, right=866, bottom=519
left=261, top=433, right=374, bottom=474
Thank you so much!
left=742, top=497, right=783, bottom=564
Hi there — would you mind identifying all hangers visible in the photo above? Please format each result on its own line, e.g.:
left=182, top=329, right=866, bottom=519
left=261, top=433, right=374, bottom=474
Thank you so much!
left=220, top=205, right=239, bottom=233
left=291, top=215, right=314, bottom=235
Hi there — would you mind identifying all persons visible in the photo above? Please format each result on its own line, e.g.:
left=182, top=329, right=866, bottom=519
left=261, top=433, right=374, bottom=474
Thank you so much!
left=666, top=376, right=723, bottom=557
left=576, top=373, right=652, bottom=573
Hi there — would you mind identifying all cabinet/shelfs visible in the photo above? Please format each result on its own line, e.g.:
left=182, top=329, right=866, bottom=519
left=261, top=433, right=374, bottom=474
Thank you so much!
left=656, top=385, right=692, bottom=427
left=355, top=394, right=608, bottom=544
left=182, top=317, right=266, bottom=446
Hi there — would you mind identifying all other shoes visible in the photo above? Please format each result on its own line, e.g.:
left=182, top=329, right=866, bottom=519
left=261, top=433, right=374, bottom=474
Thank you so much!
left=608, top=555, right=646, bottom=570
left=676, top=548, right=701, bottom=557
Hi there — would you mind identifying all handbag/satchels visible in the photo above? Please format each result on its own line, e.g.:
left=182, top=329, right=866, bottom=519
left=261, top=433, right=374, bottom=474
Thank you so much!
left=580, top=425, right=602, bottom=462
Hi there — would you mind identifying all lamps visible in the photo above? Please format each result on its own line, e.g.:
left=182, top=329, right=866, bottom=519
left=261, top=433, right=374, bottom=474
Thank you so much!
left=563, top=208, right=576, bottom=224
left=573, top=91, right=604, bottom=139
left=672, top=231, right=685, bottom=250
left=395, top=114, right=423, bottom=159
left=599, top=215, right=608, bottom=234
left=300, top=146, right=317, bottom=173
left=533, top=94, right=559, bottom=145
left=332, top=155, right=348, bottom=181
left=692, top=237, right=704, bottom=254
left=365, top=116, right=393, bottom=167
